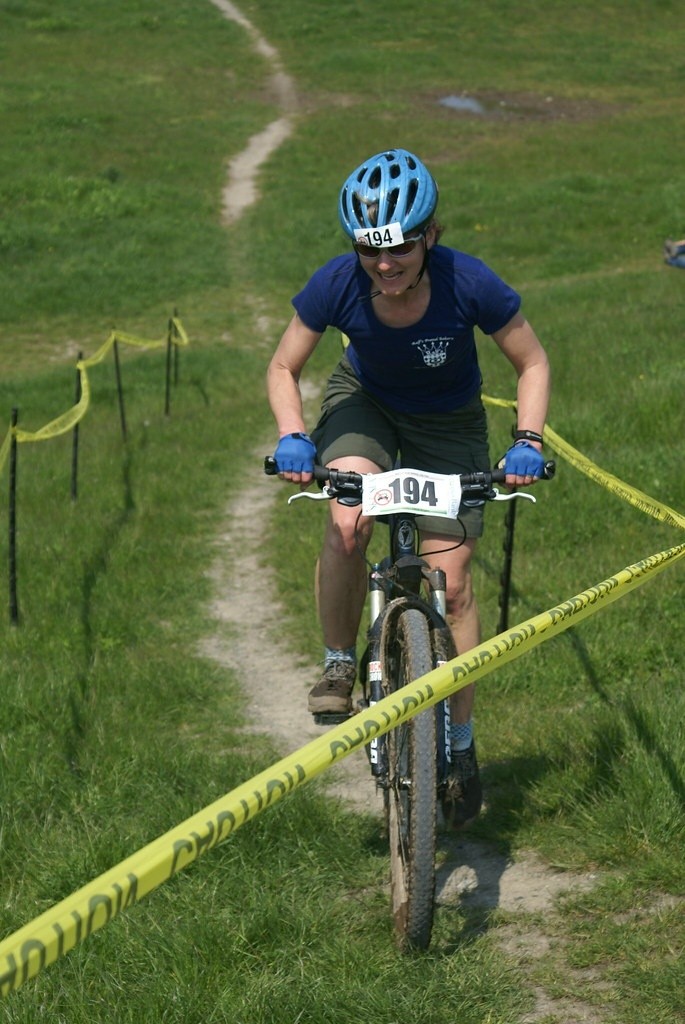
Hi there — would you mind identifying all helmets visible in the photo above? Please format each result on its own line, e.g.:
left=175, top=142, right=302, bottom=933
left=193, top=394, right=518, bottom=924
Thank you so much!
left=337, top=147, right=439, bottom=248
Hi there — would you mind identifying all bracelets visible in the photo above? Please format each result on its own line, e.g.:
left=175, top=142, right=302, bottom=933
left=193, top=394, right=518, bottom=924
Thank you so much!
left=513, top=430, right=544, bottom=444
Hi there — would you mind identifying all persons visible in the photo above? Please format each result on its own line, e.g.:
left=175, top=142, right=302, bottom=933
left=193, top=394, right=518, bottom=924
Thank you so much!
left=266, top=148, right=549, bottom=818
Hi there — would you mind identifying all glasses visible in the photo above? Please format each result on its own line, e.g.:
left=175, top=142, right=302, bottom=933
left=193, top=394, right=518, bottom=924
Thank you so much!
left=351, top=223, right=431, bottom=260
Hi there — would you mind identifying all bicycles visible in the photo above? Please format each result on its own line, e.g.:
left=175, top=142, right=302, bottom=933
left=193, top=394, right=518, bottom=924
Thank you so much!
left=263, top=453, right=555, bottom=958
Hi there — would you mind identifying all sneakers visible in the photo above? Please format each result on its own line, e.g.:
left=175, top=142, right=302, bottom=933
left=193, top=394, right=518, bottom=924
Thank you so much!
left=441, top=737, right=483, bottom=827
left=307, top=657, right=358, bottom=714
